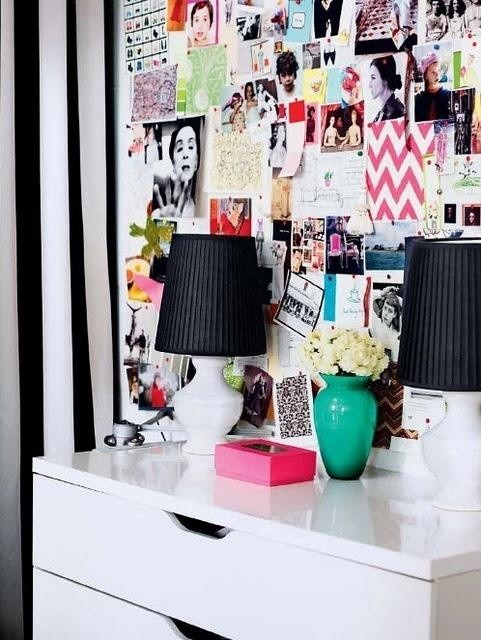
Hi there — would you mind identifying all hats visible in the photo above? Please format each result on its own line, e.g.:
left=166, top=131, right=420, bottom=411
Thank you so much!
left=307, top=106, right=315, bottom=116
left=277, top=52, right=297, bottom=72
left=421, top=53, right=438, bottom=73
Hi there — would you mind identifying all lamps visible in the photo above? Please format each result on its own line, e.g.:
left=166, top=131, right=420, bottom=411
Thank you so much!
left=396, top=237, right=481, bottom=512
left=104, top=408, right=175, bottom=449
left=153, top=232, right=267, bottom=454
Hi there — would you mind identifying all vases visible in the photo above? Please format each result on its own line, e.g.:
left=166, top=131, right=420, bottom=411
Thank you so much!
left=314, top=372, right=378, bottom=480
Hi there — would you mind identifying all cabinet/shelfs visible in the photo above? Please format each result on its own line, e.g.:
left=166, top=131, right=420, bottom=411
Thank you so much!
left=32, top=473, right=481, bottom=640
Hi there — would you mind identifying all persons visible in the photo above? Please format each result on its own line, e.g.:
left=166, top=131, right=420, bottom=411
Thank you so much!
left=129, top=372, right=168, bottom=407
left=152, top=123, right=199, bottom=218
left=465, top=210, right=479, bottom=225
left=186, top=1, right=215, bottom=46
left=370, top=293, right=401, bottom=340
left=249, top=376, right=267, bottom=417
left=445, top=206, right=456, bottom=223
left=229, top=1, right=481, bottom=169
left=132, top=329, right=146, bottom=360
left=144, top=125, right=162, bottom=163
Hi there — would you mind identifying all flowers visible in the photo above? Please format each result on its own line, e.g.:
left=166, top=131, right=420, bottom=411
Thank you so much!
left=302, top=324, right=391, bottom=382
